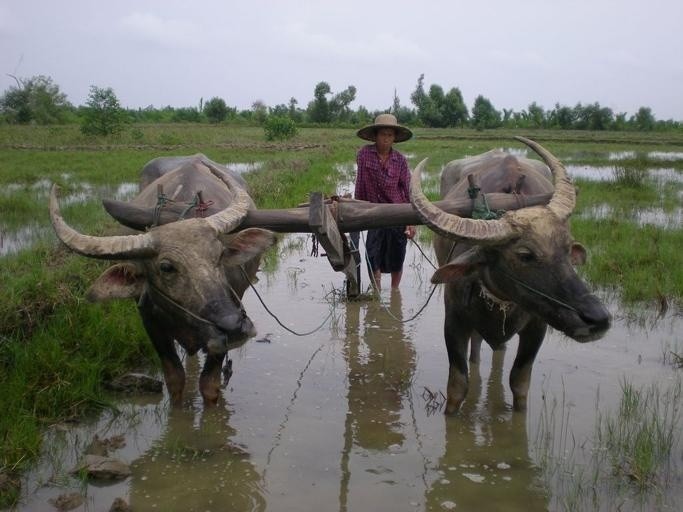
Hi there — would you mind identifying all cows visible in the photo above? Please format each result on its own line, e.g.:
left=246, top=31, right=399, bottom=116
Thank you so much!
left=47, top=154, right=276, bottom=410
left=407, top=134, right=614, bottom=415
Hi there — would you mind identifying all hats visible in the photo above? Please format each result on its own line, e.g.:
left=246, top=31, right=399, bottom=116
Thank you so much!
left=357, top=113, right=412, bottom=143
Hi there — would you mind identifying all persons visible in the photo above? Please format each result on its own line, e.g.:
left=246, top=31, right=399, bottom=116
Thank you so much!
left=354, top=113, right=416, bottom=291
left=351, top=289, right=417, bottom=452
